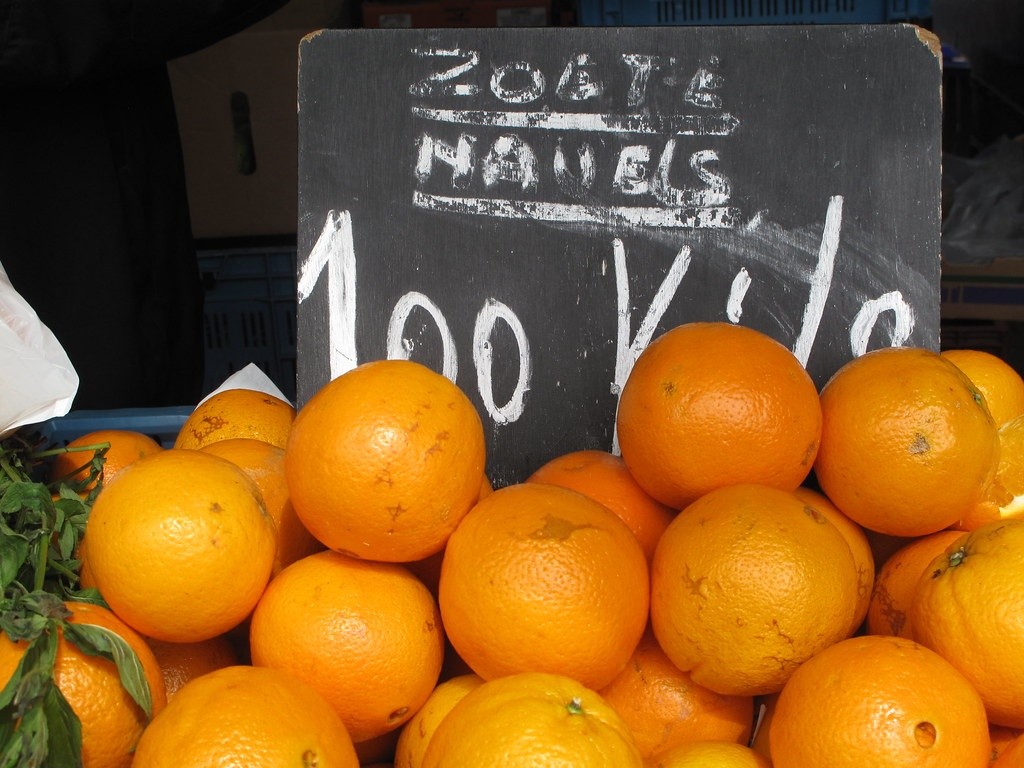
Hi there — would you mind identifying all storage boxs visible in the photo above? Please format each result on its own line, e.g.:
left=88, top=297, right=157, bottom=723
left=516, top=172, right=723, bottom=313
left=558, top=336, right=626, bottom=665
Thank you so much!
left=361, top=1, right=578, bottom=27
left=940, top=319, right=1010, bottom=364
left=166, top=1, right=354, bottom=237
left=195, top=247, right=297, bottom=405
left=940, top=258, right=1024, bottom=322
left=578, top=0, right=932, bottom=32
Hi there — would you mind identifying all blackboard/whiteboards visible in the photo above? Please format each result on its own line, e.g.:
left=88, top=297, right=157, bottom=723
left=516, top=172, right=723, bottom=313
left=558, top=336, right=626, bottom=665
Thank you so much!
left=288, top=23, right=950, bottom=491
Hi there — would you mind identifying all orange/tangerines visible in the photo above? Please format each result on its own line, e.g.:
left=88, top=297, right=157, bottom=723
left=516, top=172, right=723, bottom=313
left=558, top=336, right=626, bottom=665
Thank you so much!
left=0, top=321, right=1024, bottom=768
